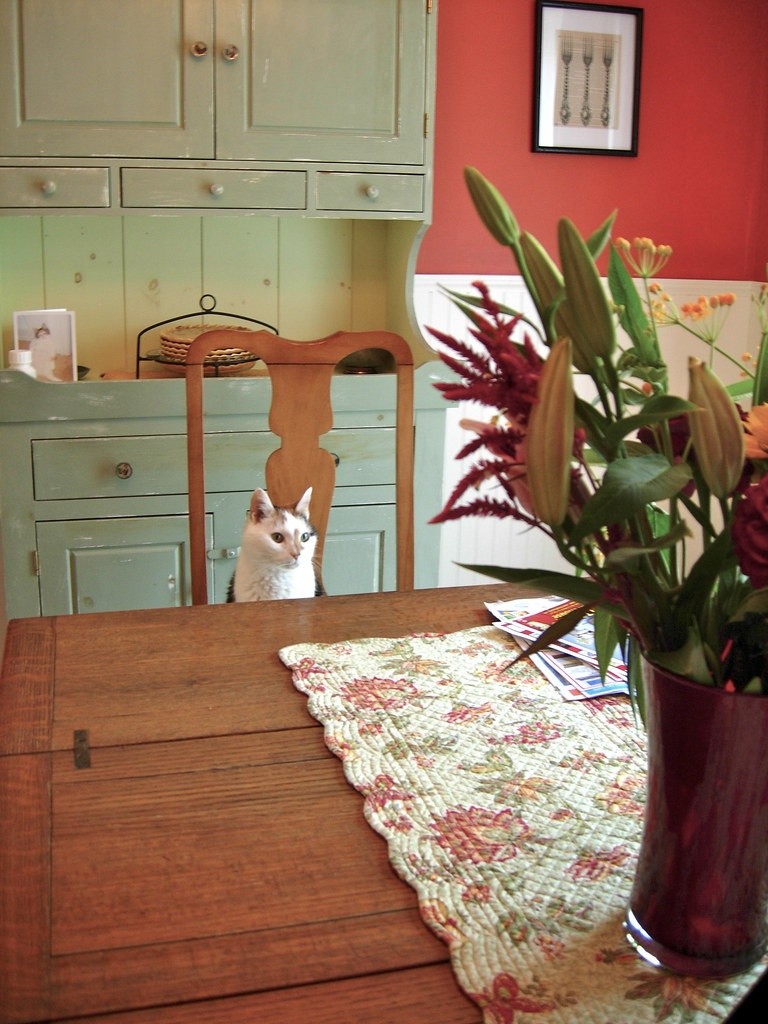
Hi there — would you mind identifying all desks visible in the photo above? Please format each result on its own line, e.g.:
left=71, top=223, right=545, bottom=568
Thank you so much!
left=0, top=577, right=768, bottom=1024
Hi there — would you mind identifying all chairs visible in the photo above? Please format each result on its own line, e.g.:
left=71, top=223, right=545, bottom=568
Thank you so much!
left=182, top=322, right=415, bottom=607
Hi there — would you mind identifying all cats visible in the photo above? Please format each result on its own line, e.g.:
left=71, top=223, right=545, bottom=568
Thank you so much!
left=226, top=486, right=328, bottom=603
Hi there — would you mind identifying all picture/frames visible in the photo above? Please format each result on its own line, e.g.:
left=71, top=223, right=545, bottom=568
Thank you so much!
left=12, top=310, right=77, bottom=382
left=529, top=0, right=644, bottom=157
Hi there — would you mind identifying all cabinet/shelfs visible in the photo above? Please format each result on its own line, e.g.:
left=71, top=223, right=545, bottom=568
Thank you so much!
left=0, top=381, right=459, bottom=636
left=0, top=0, right=435, bottom=227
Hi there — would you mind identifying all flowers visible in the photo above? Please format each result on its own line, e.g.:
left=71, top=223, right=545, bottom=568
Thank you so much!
left=429, top=154, right=768, bottom=722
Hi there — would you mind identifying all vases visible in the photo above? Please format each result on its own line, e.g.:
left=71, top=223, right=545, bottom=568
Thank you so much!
left=624, top=650, right=768, bottom=981
